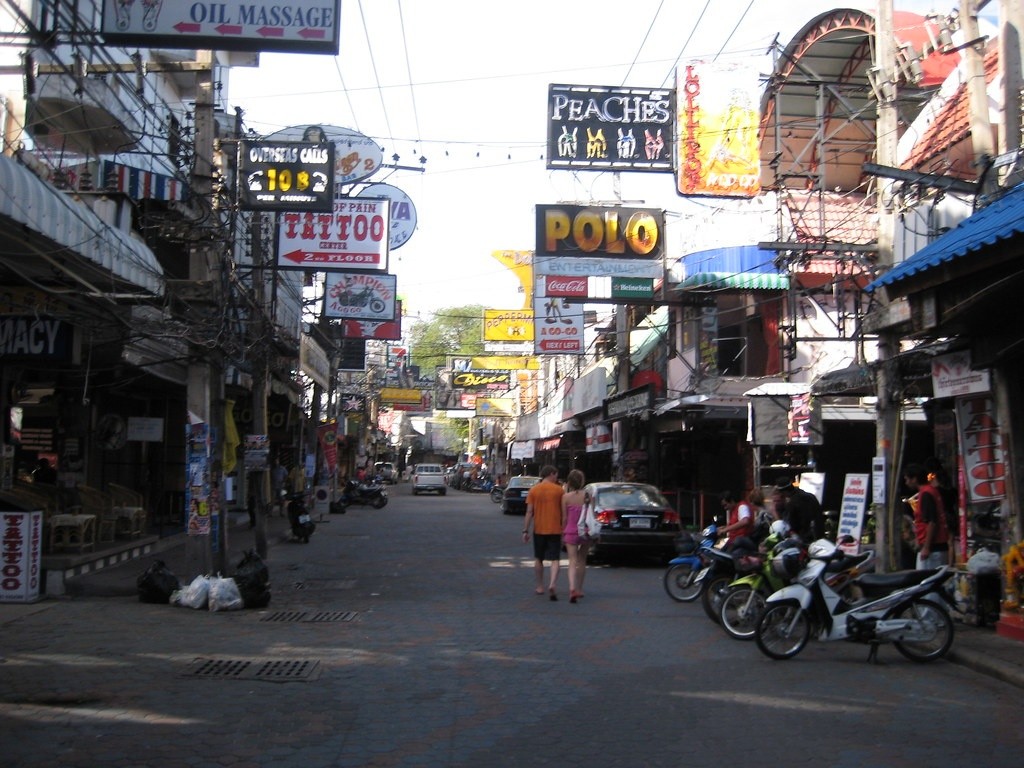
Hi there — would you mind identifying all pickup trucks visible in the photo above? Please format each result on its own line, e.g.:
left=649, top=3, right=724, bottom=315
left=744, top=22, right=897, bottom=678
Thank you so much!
left=411, top=463, right=447, bottom=496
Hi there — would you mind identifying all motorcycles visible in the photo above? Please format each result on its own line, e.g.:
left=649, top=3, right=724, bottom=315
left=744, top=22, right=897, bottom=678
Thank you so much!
left=490, top=484, right=506, bottom=503
left=339, top=477, right=388, bottom=508
left=459, top=473, right=489, bottom=491
left=663, top=512, right=736, bottom=603
left=698, top=524, right=876, bottom=640
left=753, top=531, right=966, bottom=666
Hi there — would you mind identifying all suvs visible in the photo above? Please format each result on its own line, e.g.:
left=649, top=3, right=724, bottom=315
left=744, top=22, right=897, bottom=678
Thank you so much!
left=375, top=461, right=397, bottom=482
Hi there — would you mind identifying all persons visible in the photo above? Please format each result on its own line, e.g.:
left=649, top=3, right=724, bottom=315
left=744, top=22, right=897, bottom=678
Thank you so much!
left=720, top=477, right=830, bottom=549
left=522, top=464, right=565, bottom=601
left=561, top=470, right=595, bottom=604
left=268, top=458, right=289, bottom=517
left=901, top=463, right=955, bottom=569
left=11, top=453, right=93, bottom=493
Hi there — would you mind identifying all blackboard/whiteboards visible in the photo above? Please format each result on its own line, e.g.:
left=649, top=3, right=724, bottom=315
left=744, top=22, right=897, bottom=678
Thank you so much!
left=834, top=472, right=869, bottom=554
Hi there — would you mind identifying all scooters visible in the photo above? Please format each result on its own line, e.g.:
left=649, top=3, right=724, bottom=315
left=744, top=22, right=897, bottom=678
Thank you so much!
left=281, top=483, right=316, bottom=543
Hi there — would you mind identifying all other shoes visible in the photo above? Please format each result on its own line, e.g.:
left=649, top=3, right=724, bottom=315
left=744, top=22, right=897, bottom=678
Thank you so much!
left=534, top=587, right=544, bottom=594
left=547, top=585, right=558, bottom=602
left=568, top=590, right=578, bottom=604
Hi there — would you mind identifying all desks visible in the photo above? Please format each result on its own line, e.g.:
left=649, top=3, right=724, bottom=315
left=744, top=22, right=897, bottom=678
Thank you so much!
left=47, top=514, right=96, bottom=556
left=112, top=506, right=142, bottom=541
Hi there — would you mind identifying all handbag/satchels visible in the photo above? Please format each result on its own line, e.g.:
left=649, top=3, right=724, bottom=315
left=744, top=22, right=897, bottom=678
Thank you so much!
left=576, top=492, right=602, bottom=542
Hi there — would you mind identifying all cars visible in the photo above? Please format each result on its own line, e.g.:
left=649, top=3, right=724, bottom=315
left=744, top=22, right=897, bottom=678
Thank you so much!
left=447, top=462, right=483, bottom=479
left=561, top=483, right=680, bottom=557
left=502, top=476, right=543, bottom=513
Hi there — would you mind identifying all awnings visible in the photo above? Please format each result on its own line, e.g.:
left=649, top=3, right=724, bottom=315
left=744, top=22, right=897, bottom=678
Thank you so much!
left=862, top=180, right=1024, bottom=292
left=672, top=245, right=790, bottom=293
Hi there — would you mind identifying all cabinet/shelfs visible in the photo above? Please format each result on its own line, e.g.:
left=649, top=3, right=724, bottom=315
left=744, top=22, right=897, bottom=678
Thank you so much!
left=945, top=568, right=1001, bottom=630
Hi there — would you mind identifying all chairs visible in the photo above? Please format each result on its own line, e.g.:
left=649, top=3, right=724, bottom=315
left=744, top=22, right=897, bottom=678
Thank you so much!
left=0, top=483, right=144, bottom=542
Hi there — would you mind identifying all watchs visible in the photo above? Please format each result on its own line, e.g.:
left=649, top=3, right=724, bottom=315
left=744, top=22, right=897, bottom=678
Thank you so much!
left=522, top=530, right=528, bottom=533
